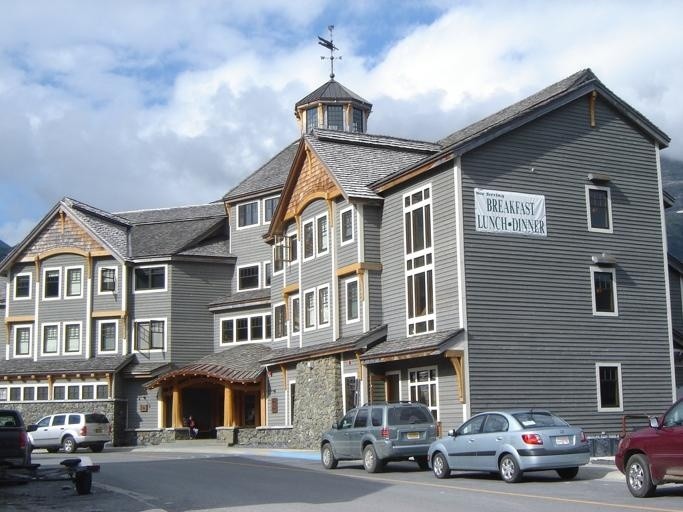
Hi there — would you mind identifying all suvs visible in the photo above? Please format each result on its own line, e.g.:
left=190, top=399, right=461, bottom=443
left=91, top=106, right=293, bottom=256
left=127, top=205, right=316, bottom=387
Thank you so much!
left=319, top=401, right=441, bottom=473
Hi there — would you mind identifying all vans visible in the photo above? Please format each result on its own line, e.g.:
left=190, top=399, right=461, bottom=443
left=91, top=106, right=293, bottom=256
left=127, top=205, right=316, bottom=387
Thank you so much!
left=28, top=411, right=114, bottom=453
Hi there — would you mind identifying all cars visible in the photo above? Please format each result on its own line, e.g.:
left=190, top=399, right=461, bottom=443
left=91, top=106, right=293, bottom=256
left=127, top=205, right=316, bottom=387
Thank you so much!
left=615, top=393, right=683, bottom=497
left=426, top=408, right=591, bottom=485
left=0, top=409, right=33, bottom=466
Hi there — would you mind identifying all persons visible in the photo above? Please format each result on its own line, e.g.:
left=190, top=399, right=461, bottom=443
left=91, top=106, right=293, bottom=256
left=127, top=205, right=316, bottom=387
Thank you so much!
left=187, top=414, right=199, bottom=438
left=181, top=415, right=192, bottom=440
